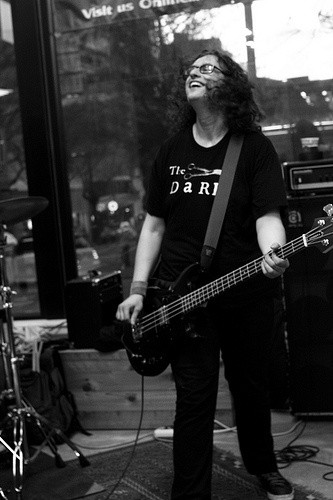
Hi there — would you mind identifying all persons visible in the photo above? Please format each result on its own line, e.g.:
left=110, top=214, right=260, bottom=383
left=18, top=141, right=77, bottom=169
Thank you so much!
left=115, top=50, right=295, bottom=500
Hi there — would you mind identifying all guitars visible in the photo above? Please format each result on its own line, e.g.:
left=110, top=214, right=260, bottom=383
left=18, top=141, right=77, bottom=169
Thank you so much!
left=123, top=204, right=333, bottom=377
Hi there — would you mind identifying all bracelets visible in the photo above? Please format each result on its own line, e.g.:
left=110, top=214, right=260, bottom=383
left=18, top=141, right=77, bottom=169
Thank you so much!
left=130, top=280, right=147, bottom=296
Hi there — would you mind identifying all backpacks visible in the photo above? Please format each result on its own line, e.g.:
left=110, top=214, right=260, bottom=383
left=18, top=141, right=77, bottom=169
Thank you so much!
left=14, top=338, right=78, bottom=441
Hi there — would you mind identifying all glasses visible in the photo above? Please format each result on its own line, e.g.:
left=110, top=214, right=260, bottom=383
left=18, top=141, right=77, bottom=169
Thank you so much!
left=182, top=64, right=227, bottom=79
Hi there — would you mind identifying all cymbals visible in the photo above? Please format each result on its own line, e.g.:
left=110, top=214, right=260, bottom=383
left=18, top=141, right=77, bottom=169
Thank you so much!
left=0, top=197, right=49, bottom=225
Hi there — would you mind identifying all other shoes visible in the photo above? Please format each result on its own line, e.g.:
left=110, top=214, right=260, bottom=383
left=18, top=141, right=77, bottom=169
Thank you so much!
left=256, top=471, right=296, bottom=500
